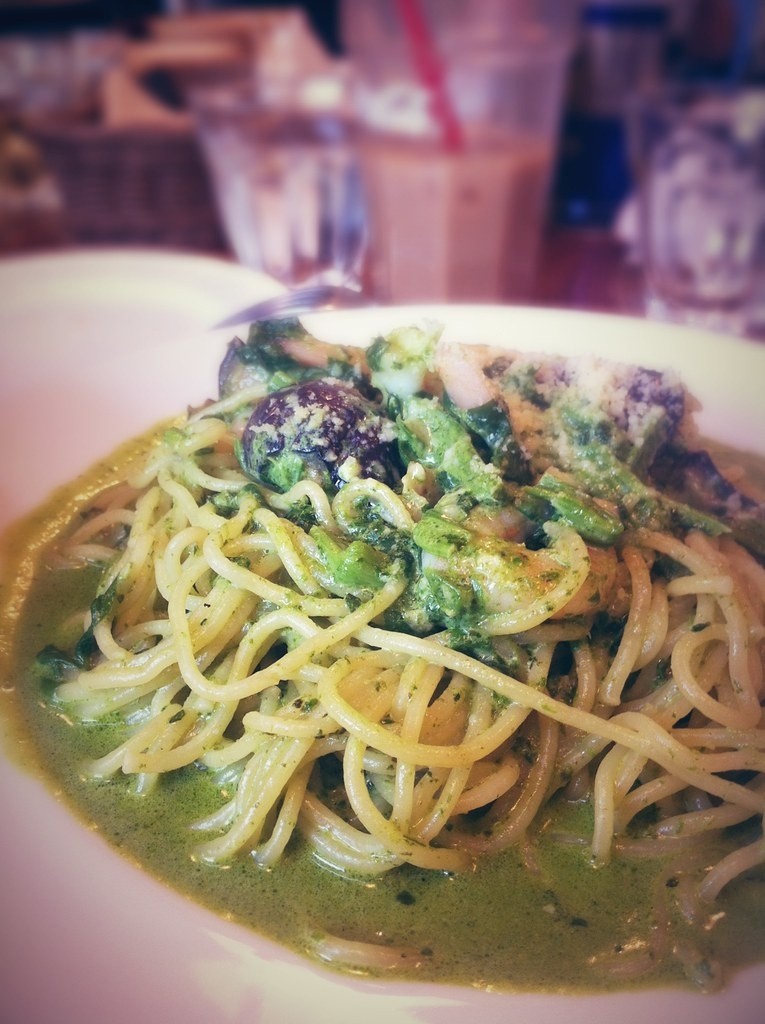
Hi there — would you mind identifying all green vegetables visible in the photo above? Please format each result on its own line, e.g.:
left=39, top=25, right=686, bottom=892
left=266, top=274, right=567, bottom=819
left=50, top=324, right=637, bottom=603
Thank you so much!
left=33, top=315, right=764, bottom=694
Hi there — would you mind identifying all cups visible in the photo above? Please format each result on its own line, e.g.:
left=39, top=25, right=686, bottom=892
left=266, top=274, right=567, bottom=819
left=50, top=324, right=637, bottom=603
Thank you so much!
left=193, top=66, right=364, bottom=306
left=361, top=47, right=565, bottom=309
left=625, top=79, right=765, bottom=333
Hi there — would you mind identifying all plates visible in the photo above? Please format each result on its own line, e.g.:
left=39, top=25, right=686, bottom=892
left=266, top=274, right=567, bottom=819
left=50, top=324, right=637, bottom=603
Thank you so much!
left=0, top=300, right=765, bottom=1024
left=0, top=249, right=305, bottom=332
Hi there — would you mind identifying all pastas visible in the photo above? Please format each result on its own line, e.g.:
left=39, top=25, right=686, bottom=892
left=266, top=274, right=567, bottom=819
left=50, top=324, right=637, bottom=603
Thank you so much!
left=33, top=372, right=765, bottom=973
